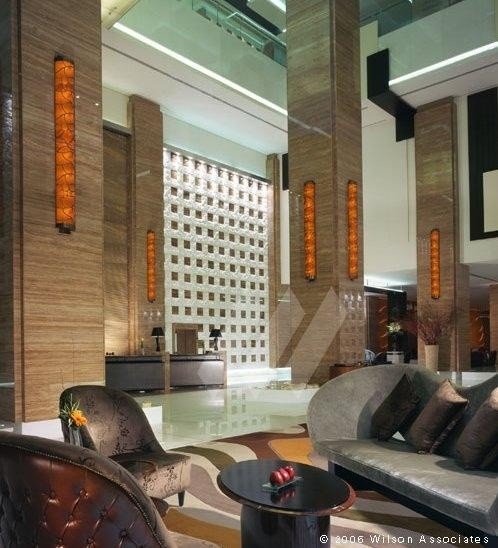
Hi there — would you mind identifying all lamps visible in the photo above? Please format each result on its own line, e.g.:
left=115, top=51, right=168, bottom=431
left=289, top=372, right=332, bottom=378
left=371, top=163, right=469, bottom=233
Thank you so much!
left=304, top=178, right=359, bottom=281
left=430, top=228, right=440, bottom=299
left=146, top=229, right=156, bottom=303
left=54, top=55, right=76, bottom=235
left=151, top=327, right=164, bottom=352
left=209, top=328, right=222, bottom=350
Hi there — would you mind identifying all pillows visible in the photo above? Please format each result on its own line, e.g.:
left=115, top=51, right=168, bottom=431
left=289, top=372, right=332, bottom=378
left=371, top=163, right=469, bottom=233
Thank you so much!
left=369, top=374, right=498, bottom=472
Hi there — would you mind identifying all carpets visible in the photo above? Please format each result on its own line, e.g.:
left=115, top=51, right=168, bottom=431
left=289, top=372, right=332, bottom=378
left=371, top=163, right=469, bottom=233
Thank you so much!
left=76, top=419, right=485, bottom=548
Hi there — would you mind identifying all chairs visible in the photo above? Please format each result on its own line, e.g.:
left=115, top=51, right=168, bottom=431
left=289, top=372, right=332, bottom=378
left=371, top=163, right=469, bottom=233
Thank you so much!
left=472, top=350, right=497, bottom=366
left=0, top=386, right=223, bottom=548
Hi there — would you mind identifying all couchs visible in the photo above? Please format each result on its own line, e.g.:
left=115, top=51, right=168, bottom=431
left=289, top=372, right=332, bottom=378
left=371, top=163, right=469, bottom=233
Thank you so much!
left=306, top=364, right=497, bottom=547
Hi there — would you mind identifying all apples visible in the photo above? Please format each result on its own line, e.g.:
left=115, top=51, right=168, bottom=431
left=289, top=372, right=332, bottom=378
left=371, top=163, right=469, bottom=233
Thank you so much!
left=270, top=466, right=294, bottom=485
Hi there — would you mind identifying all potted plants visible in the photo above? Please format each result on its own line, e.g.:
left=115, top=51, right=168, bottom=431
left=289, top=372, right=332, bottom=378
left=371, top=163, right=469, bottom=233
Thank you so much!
left=418, top=302, right=443, bottom=371
left=385, top=321, right=404, bottom=364
